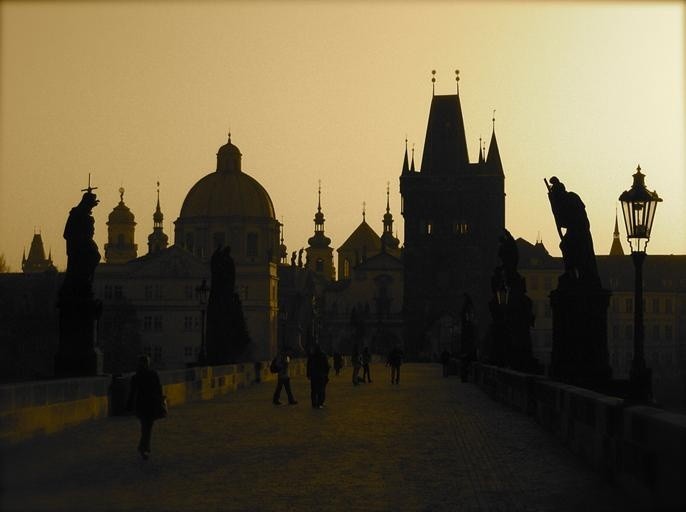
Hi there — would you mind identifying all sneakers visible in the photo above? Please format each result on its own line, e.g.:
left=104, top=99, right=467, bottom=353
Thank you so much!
left=273, top=400, right=297, bottom=405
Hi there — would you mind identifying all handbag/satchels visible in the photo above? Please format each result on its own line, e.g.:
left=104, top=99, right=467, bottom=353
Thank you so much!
left=152, top=394, right=168, bottom=420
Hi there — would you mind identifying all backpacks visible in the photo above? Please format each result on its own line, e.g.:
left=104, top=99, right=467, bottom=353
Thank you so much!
left=270, top=352, right=286, bottom=372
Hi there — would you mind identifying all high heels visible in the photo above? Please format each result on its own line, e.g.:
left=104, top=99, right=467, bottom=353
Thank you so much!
left=136, top=450, right=148, bottom=460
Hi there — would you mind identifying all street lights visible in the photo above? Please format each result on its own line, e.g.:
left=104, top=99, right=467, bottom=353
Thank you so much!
left=618, top=166, right=662, bottom=407
left=494, top=280, right=510, bottom=368
left=199, top=286, right=212, bottom=362
left=280, top=316, right=288, bottom=352
left=464, top=308, right=474, bottom=360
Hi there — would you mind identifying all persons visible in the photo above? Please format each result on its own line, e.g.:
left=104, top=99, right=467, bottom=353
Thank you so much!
left=440, top=345, right=450, bottom=378
left=334, top=350, right=344, bottom=375
left=271, top=344, right=297, bottom=404
left=361, top=346, right=373, bottom=383
left=126, top=354, right=166, bottom=457
left=385, top=342, right=404, bottom=384
left=306, top=347, right=329, bottom=409
left=352, top=351, right=362, bottom=384
